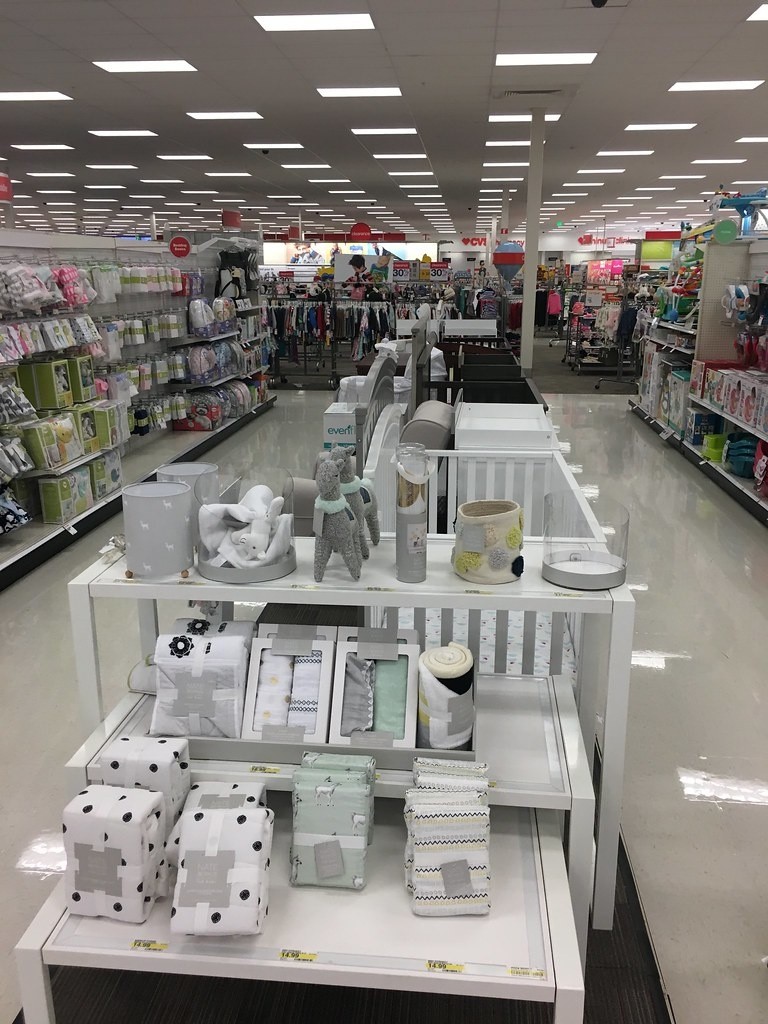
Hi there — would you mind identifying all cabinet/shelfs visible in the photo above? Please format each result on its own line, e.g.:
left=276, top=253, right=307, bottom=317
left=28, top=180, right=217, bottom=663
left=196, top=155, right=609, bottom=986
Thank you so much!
left=561, top=291, right=637, bottom=376
left=0, top=226, right=280, bottom=592
left=628, top=236, right=768, bottom=528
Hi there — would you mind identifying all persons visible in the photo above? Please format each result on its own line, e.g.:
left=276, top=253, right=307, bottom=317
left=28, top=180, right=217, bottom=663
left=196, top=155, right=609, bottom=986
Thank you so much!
left=372, top=243, right=401, bottom=261
left=733, top=381, right=741, bottom=409
left=748, top=387, right=756, bottom=416
left=560, top=259, right=564, bottom=270
left=290, top=243, right=325, bottom=264
left=330, top=243, right=342, bottom=266
left=722, top=283, right=768, bottom=371
left=479, top=260, right=487, bottom=277
left=342, top=255, right=391, bottom=292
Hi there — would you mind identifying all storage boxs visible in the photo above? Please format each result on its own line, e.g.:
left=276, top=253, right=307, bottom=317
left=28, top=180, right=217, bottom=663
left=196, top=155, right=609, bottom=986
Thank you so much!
left=18, top=363, right=41, bottom=411
left=660, top=358, right=768, bottom=445
left=23, top=412, right=84, bottom=470
left=62, top=618, right=493, bottom=937
left=65, top=355, right=98, bottom=402
left=9, top=475, right=48, bottom=517
left=85, top=448, right=123, bottom=500
left=95, top=399, right=132, bottom=447
left=61, top=403, right=100, bottom=454
left=37, top=465, right=94, bottom=524
left=34, top=359, right=74, bottom=409
left=0, top=415, right=52, bottom=446
left=86, top=399, right=107, bottom=407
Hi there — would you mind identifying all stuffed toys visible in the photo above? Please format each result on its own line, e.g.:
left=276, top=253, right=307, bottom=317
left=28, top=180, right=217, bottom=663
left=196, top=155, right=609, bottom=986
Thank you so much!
left=228, top=496, right=284, bottom=560
left=314, top=440, right=380, bottom=582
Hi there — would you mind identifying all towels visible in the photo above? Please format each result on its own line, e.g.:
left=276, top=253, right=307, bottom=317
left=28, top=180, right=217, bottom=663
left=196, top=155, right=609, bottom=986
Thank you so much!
left=288, top=750, right=492, bottom=918
left=0, top=261, right=118, bottom=312
left=60, top=736, right=276, bottom=938
left=149, top=617, right=476, bottom=751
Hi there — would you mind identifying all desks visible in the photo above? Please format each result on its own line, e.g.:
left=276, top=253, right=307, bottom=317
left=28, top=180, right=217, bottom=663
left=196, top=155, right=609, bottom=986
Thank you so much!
left=15, top=786, right=586, bottom=1024
left=69, top=533, right=636, bottom=932
left=66, top=672, right=597, bottom=979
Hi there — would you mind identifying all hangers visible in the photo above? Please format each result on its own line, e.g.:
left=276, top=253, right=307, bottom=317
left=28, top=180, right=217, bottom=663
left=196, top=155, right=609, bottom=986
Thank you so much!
left=223, top=237, right=258, bottom=251
left=266, top=299, right=457, bottom=312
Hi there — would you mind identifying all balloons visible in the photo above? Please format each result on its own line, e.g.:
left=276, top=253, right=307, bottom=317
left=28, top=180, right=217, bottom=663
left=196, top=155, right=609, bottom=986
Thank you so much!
left=493, top=242, right=525, bottom=282
